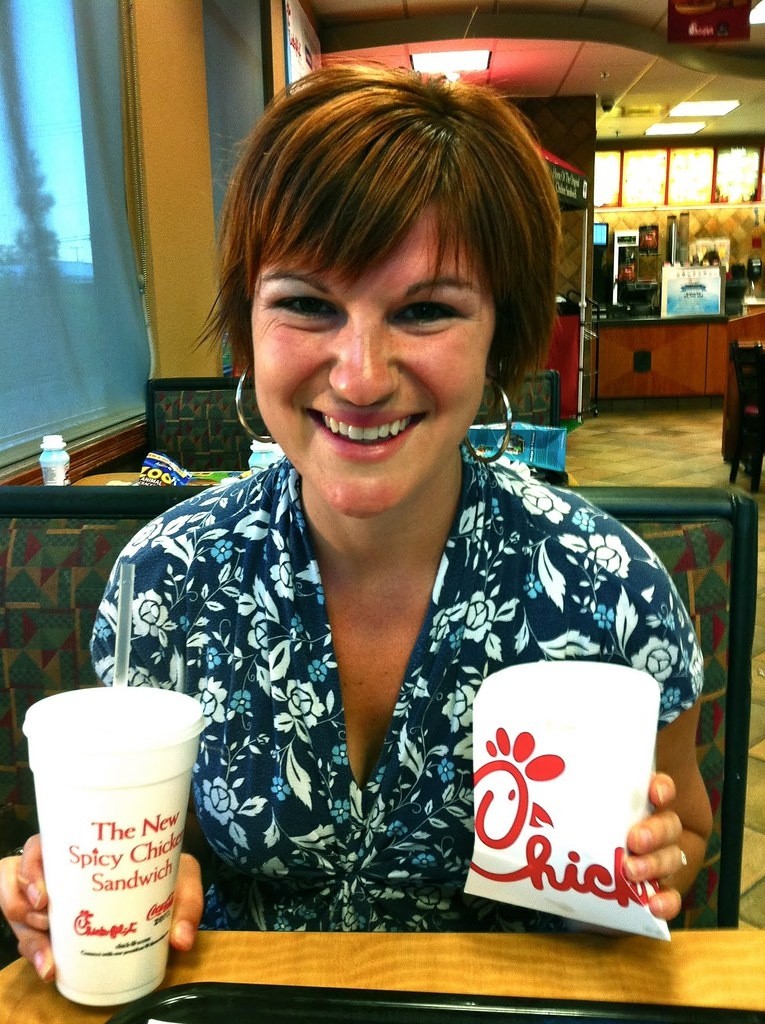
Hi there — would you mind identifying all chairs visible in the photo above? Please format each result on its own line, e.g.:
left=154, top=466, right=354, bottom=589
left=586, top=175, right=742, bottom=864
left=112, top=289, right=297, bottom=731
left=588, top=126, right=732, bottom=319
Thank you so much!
left=729, top=341, right=765, bottom=493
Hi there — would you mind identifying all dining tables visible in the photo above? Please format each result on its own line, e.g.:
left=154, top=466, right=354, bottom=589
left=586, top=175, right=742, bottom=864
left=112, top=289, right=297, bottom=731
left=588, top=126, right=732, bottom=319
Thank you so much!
left=0, top=930, right=763, bottom=1024
left=68, top=469, right=257, bottom=486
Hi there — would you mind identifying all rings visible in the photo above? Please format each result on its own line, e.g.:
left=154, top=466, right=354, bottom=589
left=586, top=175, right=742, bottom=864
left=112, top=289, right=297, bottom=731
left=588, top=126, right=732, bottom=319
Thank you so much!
left=660, top=850, right=688, bottom=885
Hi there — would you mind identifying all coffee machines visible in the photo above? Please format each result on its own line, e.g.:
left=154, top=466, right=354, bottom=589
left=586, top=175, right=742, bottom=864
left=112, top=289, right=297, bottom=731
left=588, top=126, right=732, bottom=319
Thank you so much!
left=612, top=225, right=660, bottom=311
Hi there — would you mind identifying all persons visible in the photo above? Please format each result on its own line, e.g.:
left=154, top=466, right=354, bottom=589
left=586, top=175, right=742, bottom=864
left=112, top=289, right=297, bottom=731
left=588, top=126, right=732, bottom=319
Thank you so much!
left=0, top=60, right=716, bottom=989
left=700, top=250, right=732, bottom=282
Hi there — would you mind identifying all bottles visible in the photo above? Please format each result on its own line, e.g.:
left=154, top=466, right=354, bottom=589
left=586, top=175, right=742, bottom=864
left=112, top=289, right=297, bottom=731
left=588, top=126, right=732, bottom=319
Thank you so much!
left=247, top=435, right=276, bottom=478
left=39, top=434, right=71, bottom=487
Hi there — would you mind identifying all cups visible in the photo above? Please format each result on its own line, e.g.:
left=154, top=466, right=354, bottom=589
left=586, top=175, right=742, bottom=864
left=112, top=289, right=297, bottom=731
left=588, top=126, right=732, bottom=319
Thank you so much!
left=20, top=690, right=207, bottom=1009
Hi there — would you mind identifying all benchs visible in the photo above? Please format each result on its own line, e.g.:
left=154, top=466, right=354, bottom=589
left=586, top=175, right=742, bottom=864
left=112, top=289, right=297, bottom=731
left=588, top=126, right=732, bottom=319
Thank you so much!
left=1, top=482, right=761, bottom=958
left=144, top=368, right=560, bottom=472
